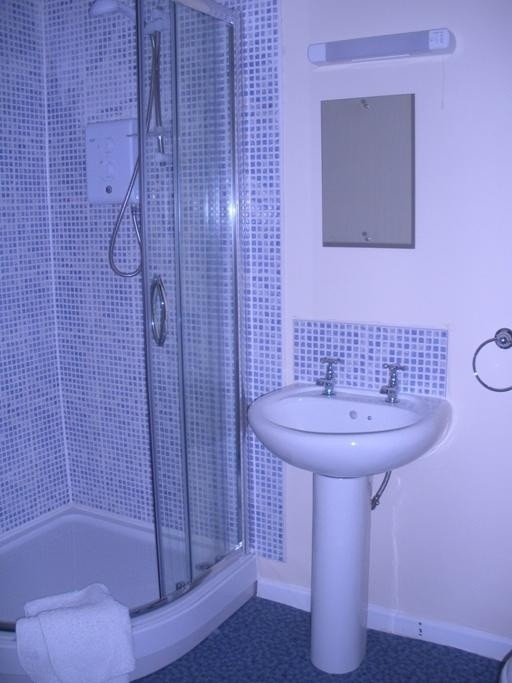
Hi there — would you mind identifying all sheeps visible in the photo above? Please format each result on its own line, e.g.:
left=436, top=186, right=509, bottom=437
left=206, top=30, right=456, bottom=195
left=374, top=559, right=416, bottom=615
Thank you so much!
left=379, top=363, right=406, bottom=406
left=315, top=357, right=343, bottom=395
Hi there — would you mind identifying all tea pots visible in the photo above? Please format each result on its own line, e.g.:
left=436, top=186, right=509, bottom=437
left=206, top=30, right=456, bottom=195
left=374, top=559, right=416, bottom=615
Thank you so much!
left=320, top=94, right=415, bottom=248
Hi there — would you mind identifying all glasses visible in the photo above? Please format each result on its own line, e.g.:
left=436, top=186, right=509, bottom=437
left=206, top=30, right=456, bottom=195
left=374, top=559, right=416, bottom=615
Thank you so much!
left=308, top=27, right=456, bottom=66
